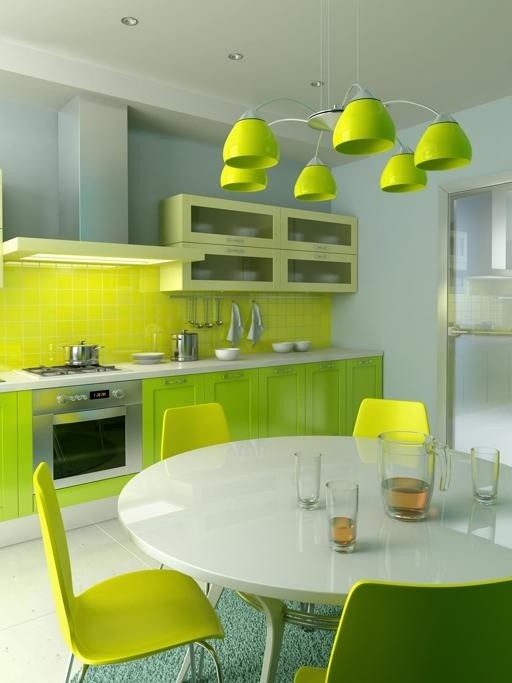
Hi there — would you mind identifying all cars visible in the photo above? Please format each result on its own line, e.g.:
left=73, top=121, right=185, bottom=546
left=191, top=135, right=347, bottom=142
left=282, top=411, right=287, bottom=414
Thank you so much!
left=378, top=431, right=451, bottom=522
left=294, top=451, right=321, bottom=510
left=471, top=447, right=499, bottom=508
left=325, top=480, right=358, bottom=554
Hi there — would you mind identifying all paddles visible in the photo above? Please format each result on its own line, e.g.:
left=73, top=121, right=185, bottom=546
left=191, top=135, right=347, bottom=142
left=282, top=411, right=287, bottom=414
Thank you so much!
left=70, top=587, right=344, bottom=683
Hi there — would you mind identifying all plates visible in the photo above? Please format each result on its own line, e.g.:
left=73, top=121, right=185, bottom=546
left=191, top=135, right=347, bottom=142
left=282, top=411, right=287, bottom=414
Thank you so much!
left=455, top=336, right=512, bottom=411
left=258, top=352, right=305, bottom=439
left=280, top=205, right=357, bottom=255
left=160, top=193, right=281, bottom=248
left=280, top=250, right=358, bottom=293
left=159, top=242, right=280, bottom=294
left=105, top=358, right=205, bottom=475
left=346, top=348, right=383, bottom=436
left=305, top=346, right=346, bottom=444
left=1, top=370, right=34, bottom=549
left=204, top=356, right=259, bottom=441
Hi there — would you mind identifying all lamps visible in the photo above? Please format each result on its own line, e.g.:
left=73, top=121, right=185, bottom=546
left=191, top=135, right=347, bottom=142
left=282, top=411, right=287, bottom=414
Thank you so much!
left=33, top=460, right=226, bottom=683
left=353, top=397, right=431, bottom=445
left=160, top=402, right=232, bottom=596
left=294, top=579, right=512, bottom=683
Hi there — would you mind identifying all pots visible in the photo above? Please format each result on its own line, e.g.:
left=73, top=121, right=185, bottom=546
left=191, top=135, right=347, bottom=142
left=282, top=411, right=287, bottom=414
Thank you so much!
left=170, top=329, right=198, bottom=362
left=63, top=340, right=104, bottom=366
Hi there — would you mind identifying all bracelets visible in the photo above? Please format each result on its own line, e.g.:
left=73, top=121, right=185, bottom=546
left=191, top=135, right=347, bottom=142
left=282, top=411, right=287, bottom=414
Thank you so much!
left=215, top=348, right=240, bottom=360
left=272, top=342, right=294, bottom=353
left=131, top=352, right=165, bottom=365
left=293, top=341, right=312, bottom=352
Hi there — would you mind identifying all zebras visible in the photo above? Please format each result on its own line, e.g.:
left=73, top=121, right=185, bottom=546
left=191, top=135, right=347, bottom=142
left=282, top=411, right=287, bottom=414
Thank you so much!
left=32, top=380, right=143, bottom=494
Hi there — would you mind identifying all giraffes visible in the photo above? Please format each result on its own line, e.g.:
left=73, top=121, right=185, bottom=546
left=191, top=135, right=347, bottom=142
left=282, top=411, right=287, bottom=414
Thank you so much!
left=22, top=364, right=122, bottom=377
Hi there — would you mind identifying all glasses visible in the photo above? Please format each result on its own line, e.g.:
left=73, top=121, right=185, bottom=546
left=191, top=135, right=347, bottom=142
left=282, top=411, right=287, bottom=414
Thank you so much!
left=220, top=84, right=472, bottom=201
